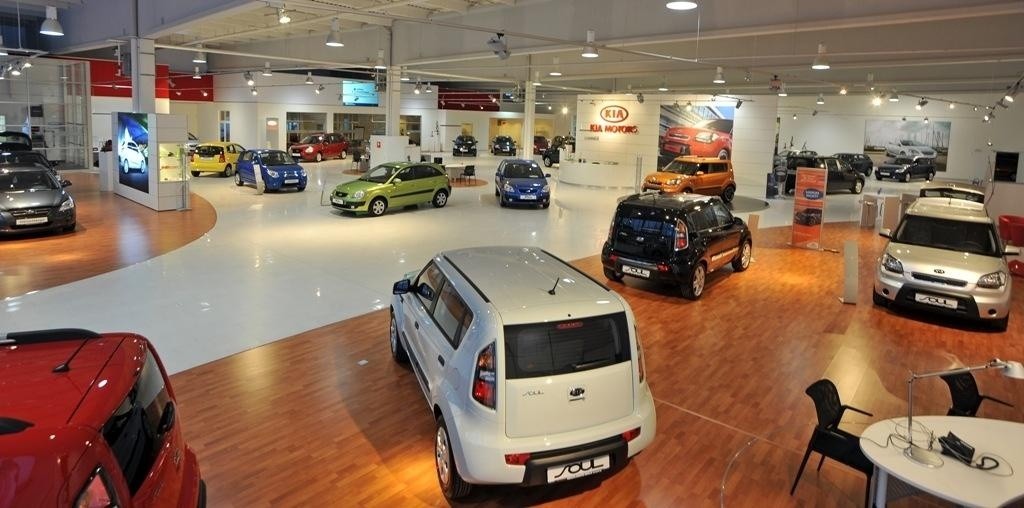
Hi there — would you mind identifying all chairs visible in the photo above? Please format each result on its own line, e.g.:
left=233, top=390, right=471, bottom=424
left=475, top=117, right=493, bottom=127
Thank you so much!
left=938, top=368, right=1015, bottom=417
left=790, top=378, right=874, bottom=508
left=434, top=157, right=442, bottom=164
left=351, top=151, right=361, bottom=172
left=460, top=165, right=476, bottom=184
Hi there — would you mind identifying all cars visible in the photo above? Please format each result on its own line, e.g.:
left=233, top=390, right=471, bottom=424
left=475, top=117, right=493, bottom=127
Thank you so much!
left=0, top=130, right=76, bottom=238
left=884, top=138, right=937, bottom=163
left=495, top=158, right=554, bottom=211
left=451, top=131, right=575, bottom=168
left=775, top=148, right=936, bottom=195
left=874, top=183, right=1018, bottom=330
left=186, top=128, right=350, bottom=195
left=330, top=159, right=452, bottom=219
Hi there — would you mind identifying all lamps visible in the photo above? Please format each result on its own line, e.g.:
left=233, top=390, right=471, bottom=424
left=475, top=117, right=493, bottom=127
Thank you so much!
left=903, top=357, right=1024, bottom=468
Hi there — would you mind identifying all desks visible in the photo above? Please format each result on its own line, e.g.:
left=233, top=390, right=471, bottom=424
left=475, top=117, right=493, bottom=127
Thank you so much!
left=859, top=414, right=1024, bottom=508
left=445, top=165, right=465, bottom=182
left=360, top=157, right=369, bottom=172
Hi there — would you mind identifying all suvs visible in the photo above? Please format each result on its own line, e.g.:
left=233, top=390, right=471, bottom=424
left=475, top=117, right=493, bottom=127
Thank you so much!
left=389, top=247, right=657, bottom=502
left=638, top=152, right=735, bottom=205
left=662, top=118, right=733, bottom=161
left=601, top=191, right=752, bottom=302
left=0, top=325, right=208, bottom=507
